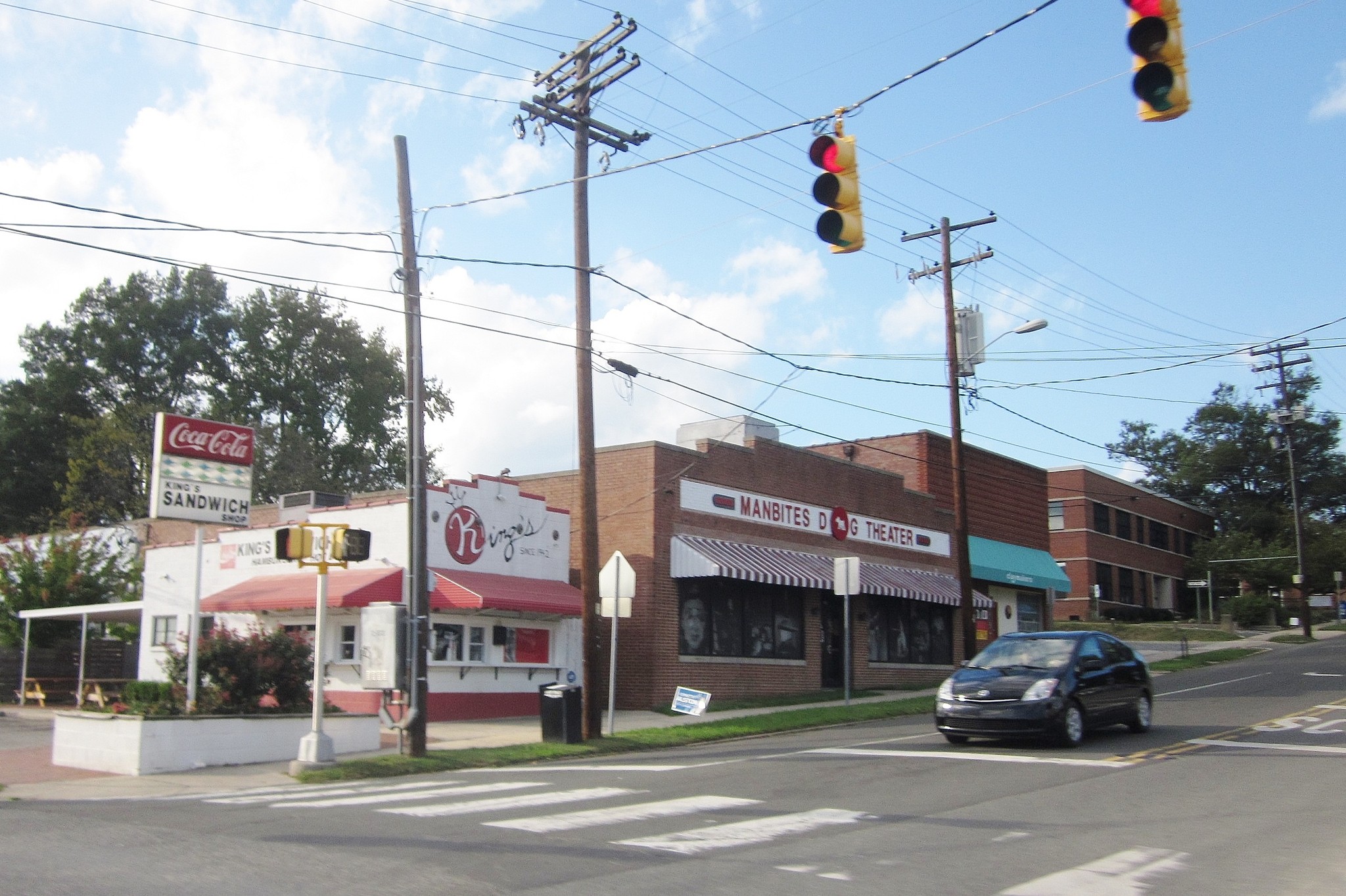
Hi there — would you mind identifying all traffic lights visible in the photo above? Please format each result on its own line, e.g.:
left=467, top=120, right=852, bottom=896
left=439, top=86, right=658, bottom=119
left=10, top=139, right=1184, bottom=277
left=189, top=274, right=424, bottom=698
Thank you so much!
left=808, top=133, right=864, bottom=253
left=275, top=527, right=313, bottom=561
left=1122, top=0, right=1189, bottom=124
left=330, top=527, right=371, bottom=561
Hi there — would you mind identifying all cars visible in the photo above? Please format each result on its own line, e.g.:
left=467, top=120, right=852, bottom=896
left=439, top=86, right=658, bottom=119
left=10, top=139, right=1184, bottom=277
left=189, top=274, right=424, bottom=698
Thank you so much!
left=933, top=630, right=1155, bottom=746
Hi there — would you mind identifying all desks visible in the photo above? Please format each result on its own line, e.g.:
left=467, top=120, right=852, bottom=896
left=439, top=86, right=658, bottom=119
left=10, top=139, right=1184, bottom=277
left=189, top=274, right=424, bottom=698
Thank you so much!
left=22, top=676, right=77, bottom=707
left=79, top=678, right=136, bottom=709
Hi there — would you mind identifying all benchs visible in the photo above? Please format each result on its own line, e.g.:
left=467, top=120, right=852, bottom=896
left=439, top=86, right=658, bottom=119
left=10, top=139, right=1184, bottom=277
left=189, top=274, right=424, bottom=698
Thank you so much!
left=14, top=690, right=68, bottom=698
left=69, top=691, right=122, bottom=698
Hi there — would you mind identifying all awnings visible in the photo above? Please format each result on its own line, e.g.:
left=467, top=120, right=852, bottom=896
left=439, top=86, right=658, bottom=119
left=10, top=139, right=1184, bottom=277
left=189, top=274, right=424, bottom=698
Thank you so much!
left=423, top=565, right=583, bottom=619
left=967, top=535, right=1072, bottom=594
left=669, top=534, right=993, bottom=608
left=198, top=567, right=404, bottom=612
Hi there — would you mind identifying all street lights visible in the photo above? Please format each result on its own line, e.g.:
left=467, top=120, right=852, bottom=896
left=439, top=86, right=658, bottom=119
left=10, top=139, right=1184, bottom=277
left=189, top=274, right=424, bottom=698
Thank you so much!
left=943, top=319, right=1048, bottom=664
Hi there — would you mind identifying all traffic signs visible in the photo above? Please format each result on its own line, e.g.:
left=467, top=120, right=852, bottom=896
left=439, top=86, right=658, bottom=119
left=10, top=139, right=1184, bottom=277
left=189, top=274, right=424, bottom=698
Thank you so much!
left=1186, top=579, right=1210, bottom=588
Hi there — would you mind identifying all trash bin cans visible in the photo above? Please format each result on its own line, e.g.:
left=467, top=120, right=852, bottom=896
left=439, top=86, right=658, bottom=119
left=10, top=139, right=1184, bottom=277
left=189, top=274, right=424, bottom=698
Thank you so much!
left=539, top=681, right=583, bottom=744
